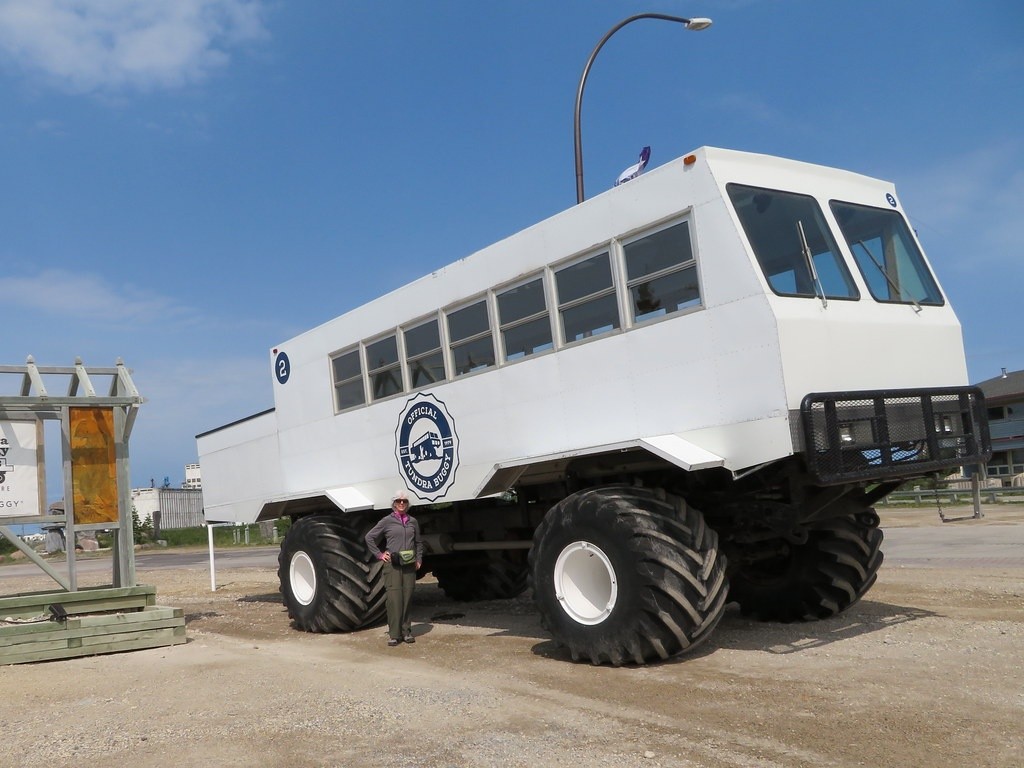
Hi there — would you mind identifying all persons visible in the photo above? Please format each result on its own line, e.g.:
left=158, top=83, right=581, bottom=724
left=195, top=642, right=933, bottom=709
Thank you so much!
left=364, top=493, right=423, bottom=647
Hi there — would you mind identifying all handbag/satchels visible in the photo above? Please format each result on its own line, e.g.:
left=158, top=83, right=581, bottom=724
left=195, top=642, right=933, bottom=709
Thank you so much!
left=399, top=550, right=417, bottom=565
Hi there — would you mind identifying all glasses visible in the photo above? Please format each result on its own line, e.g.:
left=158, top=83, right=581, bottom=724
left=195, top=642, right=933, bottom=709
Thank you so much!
left=394, top=499, right=409, bottom=503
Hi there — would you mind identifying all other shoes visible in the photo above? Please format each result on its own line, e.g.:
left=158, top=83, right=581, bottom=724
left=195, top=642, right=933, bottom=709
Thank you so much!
left=388, top=638, right=398, bottom=646
left=403, top=633, right=415, bottom=643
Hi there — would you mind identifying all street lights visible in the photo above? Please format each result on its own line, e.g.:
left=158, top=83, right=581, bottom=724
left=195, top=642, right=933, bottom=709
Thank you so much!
left=574, top=12, right=713, bottom=204
